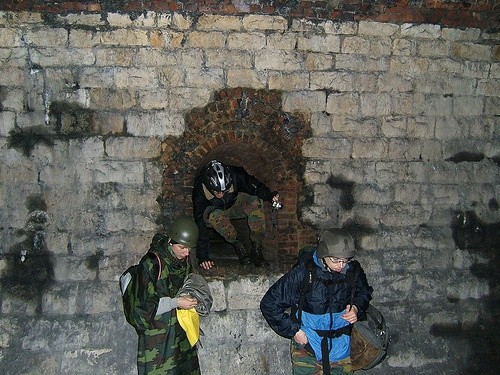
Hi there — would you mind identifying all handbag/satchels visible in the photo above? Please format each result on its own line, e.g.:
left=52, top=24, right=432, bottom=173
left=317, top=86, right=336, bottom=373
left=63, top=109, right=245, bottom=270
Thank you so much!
left=350, top=303, right=390, bottom=370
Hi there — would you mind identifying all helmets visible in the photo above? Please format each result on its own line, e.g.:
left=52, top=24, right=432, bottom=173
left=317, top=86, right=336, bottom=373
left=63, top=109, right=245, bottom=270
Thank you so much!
left=167, top=216, right=200, bottom=248
left=317, top=228, right=356, bottom=260
left=204, top=159, right=233, bottom=192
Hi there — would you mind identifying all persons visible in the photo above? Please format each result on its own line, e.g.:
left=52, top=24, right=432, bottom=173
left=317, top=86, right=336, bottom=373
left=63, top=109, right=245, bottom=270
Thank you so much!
left=134, top=218, right=201, bottom=375
left=259, top=228, right=372, bottom=375
left=191, top=160, right=280, bottom=269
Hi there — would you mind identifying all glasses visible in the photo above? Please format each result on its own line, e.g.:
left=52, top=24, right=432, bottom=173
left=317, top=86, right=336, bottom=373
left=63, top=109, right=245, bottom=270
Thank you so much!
left=329, top=257, right=353, bottom=264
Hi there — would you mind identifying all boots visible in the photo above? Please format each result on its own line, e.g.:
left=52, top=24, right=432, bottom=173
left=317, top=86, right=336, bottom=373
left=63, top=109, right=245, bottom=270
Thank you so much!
left=235, top=240, right=251, bottom=264
left=251, top=241, right=270, bottom=267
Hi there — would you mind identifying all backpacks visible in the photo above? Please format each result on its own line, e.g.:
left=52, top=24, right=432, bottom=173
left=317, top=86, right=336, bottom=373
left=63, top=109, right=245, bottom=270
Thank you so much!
left=119, top=250, right=161, bottom=335
